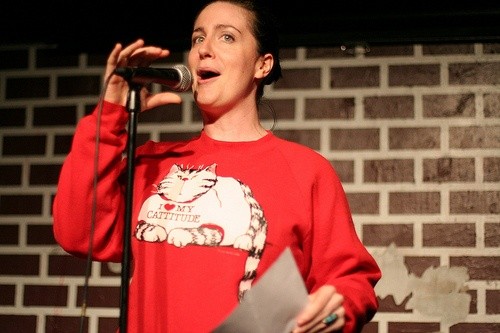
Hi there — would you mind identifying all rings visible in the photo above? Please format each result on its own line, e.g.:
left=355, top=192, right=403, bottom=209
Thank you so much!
left=323, top=313, right=338, bottom=325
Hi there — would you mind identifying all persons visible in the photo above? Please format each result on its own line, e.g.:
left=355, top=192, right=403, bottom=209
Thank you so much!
left=53, top=0, right=381, bottom=333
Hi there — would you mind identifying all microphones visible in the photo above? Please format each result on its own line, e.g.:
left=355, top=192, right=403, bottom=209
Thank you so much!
left=114, top=64, right=192, bottom=93
